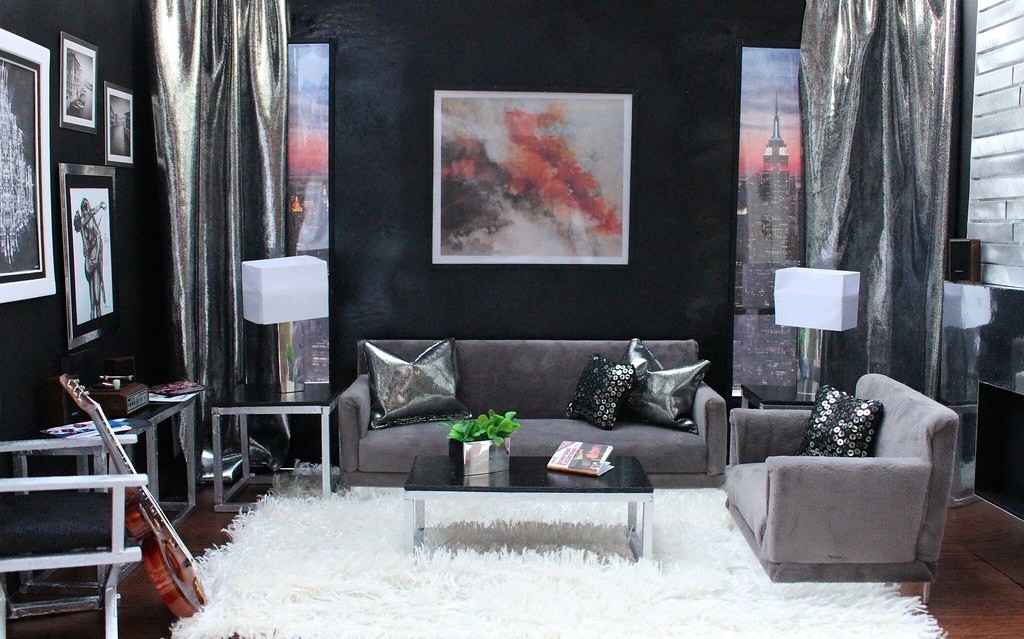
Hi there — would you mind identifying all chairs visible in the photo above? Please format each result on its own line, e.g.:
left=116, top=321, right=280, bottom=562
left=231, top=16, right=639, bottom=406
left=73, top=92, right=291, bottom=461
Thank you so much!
left=0, top=434, right=149, bottom=639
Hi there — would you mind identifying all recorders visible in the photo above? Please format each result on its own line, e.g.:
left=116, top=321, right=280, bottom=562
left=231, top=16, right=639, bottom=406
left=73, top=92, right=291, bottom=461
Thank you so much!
left=87, top=381, right=150, bottom=418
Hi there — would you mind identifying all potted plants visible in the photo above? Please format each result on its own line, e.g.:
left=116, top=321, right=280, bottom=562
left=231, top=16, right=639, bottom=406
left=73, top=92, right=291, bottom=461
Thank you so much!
left=437, top=408, right=520, bottom=476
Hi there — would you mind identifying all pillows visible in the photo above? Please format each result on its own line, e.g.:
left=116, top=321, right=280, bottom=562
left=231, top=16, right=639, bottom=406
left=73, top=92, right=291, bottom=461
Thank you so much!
left=796, top=385, right=884, bottom=458
left=619, top=338, right=711, bottom=433
left=564, top=353, right=636, bottom=430
left=364, top=337, right=472, bottom=430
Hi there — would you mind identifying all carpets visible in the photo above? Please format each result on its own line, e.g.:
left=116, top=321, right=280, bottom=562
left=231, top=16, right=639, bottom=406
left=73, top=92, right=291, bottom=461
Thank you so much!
left=162, top=459, right=948, bottom=639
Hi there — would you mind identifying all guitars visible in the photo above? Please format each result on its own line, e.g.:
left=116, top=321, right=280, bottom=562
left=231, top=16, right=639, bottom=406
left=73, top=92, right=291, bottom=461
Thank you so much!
left=58, top=374, right=208, bottom=617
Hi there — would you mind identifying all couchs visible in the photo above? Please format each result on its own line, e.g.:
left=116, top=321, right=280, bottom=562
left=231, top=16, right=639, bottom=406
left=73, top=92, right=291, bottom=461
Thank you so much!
left=339, top=340, right=726, bottom=488
left=723, top=373, right=959, bottom=604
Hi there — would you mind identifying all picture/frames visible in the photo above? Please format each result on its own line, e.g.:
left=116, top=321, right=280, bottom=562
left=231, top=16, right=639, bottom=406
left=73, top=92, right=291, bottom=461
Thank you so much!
left=104, top=80, right=134, bottom=168
left=432, top=91, right=632, bottom=266
left=0, top=28, right=56, bottom=304
left=58, top=163, right=119, bottom=350
left=59, top=31, right=98, bottom=135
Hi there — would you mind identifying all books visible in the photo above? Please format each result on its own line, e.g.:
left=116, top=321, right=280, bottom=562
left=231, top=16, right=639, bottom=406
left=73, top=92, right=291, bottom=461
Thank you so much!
left=547, top=439, right=616, bottom=477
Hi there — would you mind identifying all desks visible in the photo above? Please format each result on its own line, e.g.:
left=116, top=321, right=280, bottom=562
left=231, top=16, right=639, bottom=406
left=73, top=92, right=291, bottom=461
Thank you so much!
left=211, top=384, right=345, bottom=513
left=76, top=391, right=200, bottom=595
left=404, top=454, right=654, bottom=563
left=741, top=385, right=820, bottom=409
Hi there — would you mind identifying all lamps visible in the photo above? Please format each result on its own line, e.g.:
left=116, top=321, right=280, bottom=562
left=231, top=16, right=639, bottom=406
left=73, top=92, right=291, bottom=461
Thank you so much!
left=774, top=267, right=861, bottom=395
left=241, top=255, right=329, bottom=393
